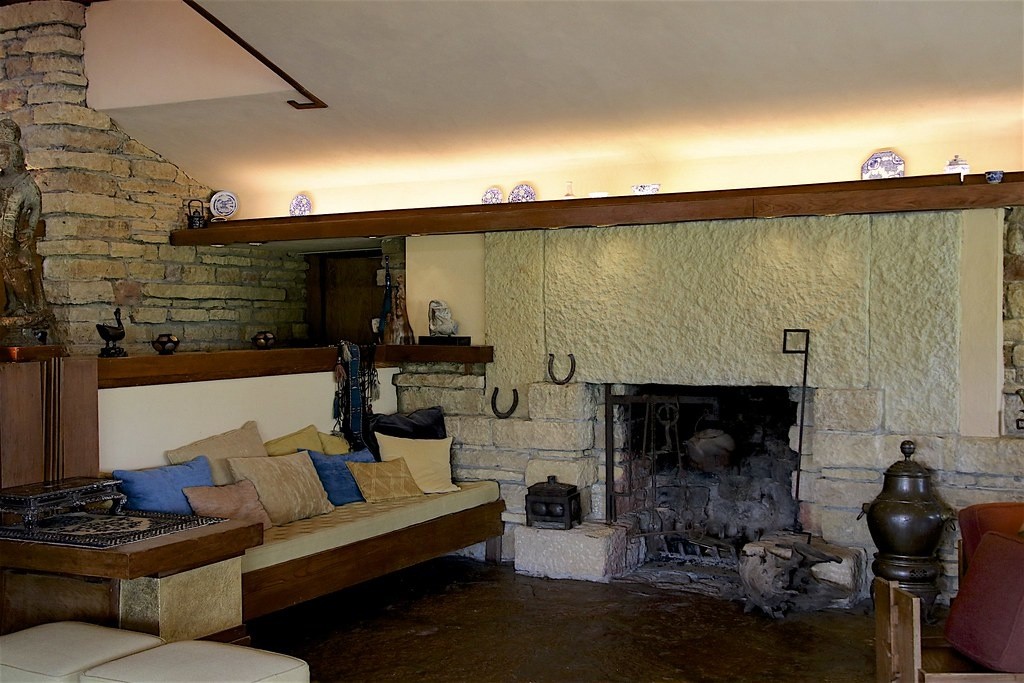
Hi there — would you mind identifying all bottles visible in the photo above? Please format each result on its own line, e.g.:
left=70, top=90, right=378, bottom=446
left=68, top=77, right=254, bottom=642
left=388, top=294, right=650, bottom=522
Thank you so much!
left=944, top=155, right=969, bottom=181
left=563, top=181, right=574, bottom=200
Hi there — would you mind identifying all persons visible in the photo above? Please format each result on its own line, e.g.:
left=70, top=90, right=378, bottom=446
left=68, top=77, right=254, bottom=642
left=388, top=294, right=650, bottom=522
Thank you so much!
left=0, top=119, right=42, bottom=316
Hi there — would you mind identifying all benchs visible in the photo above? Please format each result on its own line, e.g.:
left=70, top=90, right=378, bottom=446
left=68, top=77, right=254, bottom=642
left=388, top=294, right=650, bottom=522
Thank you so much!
left=0, top=621, right=310, bottom=683
left=242, top=480, right=506, bottom=647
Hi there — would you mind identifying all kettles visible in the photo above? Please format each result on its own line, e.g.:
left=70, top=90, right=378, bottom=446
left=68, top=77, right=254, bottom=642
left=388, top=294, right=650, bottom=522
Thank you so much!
left=681, top=428, right=735, bottom=466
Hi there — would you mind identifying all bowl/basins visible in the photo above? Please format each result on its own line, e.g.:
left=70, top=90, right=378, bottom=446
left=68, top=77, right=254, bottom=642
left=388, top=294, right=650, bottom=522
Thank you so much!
left=631, top=184, right=661, bottom=195
left=985, top=170, right=1003, bottom=184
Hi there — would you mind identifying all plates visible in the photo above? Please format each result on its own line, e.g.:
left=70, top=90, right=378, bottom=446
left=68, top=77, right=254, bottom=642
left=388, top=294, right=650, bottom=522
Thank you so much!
left=861, top=150, right=905, bottom=180
left=210, top=191, right=238, bottom=219
left=482, top=188, right=503, bottom=204
left=289, top=194, right=312, bottom=216
left=507, top=184, right=536, bottom=203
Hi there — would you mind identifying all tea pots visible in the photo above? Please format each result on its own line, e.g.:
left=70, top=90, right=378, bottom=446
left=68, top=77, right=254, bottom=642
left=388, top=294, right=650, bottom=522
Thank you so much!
left=185, top=199, right=205, bottom=229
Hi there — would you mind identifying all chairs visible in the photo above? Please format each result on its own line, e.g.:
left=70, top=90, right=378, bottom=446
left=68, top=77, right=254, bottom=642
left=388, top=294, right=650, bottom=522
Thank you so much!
left=874, top=577, right=1024, bottom=683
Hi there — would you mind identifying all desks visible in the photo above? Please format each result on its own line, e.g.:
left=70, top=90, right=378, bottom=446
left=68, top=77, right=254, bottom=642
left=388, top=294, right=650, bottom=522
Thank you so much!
left=0, top=511, right=263, bottom=647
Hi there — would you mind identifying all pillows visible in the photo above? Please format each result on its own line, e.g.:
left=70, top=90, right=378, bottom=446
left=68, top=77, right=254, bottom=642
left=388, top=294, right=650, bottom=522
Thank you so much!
left=344, top=457, right=425, bottom=503
left=167, top=421, right=269, bottom=487
left=112, top=455, right=214, bottom=517
left=225, top=450, right=335, bottom=525
left=367, top=405, right=447, bottom=463
left=182, top=479, right=272, bottom=532
left=942, top=501, right=1024, bottom=674
left=318, top=432, right=350, bottom=455
left=297, top=446, right=377, bottom=506
left=373, top=432, right=461, bottom=494
left=264, top=424, right=324, bottom=457
